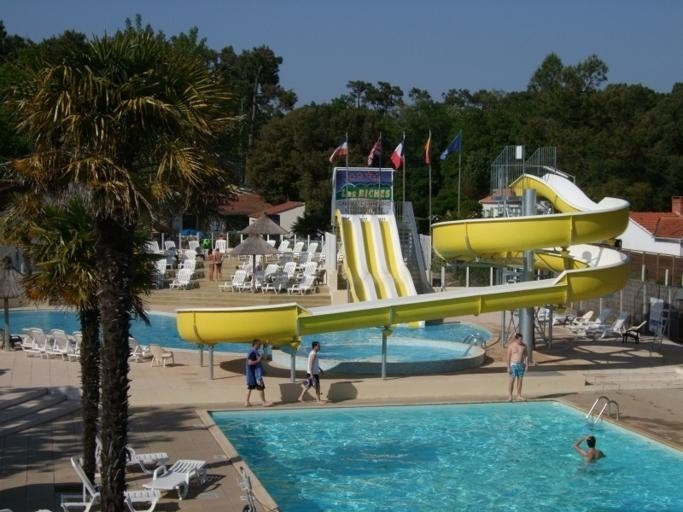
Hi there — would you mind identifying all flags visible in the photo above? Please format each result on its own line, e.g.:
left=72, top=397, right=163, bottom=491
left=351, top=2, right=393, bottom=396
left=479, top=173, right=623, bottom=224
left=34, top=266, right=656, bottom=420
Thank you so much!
left=390, top=137, right=406, bottom=170
left=439, top=134, right=462, bottom=161
left=328, top=137, right=349, bottom=164
left=424, top=136, right=431, bottom=164
left=367, top=136, right=382, bottom=167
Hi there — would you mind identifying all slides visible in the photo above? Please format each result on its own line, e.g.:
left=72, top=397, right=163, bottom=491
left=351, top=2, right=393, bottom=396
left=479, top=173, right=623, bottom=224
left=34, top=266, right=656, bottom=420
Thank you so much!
left=176, top=172, right=631, bottom=342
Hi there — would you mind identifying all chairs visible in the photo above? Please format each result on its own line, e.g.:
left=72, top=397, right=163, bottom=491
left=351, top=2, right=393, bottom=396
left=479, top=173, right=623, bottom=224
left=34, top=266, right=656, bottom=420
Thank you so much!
left=144, top=239, right=347, bottom=297
left=551, top=306, right=649, bottom=344
left=19, top=326, right=175, bottom=369
left=59, top=432, right=208, bottom=511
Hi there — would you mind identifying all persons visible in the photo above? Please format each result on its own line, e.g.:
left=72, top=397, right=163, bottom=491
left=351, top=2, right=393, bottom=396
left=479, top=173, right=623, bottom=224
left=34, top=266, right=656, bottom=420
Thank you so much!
left=206, top=248, right=227, bottom=282
left=507, top=333, right=529, bottom=401
left=574, top=435, right=606, bottom=465
left=245, top=340, right=273, bottom=407
left=297, top=341, right=328, bottom=405
